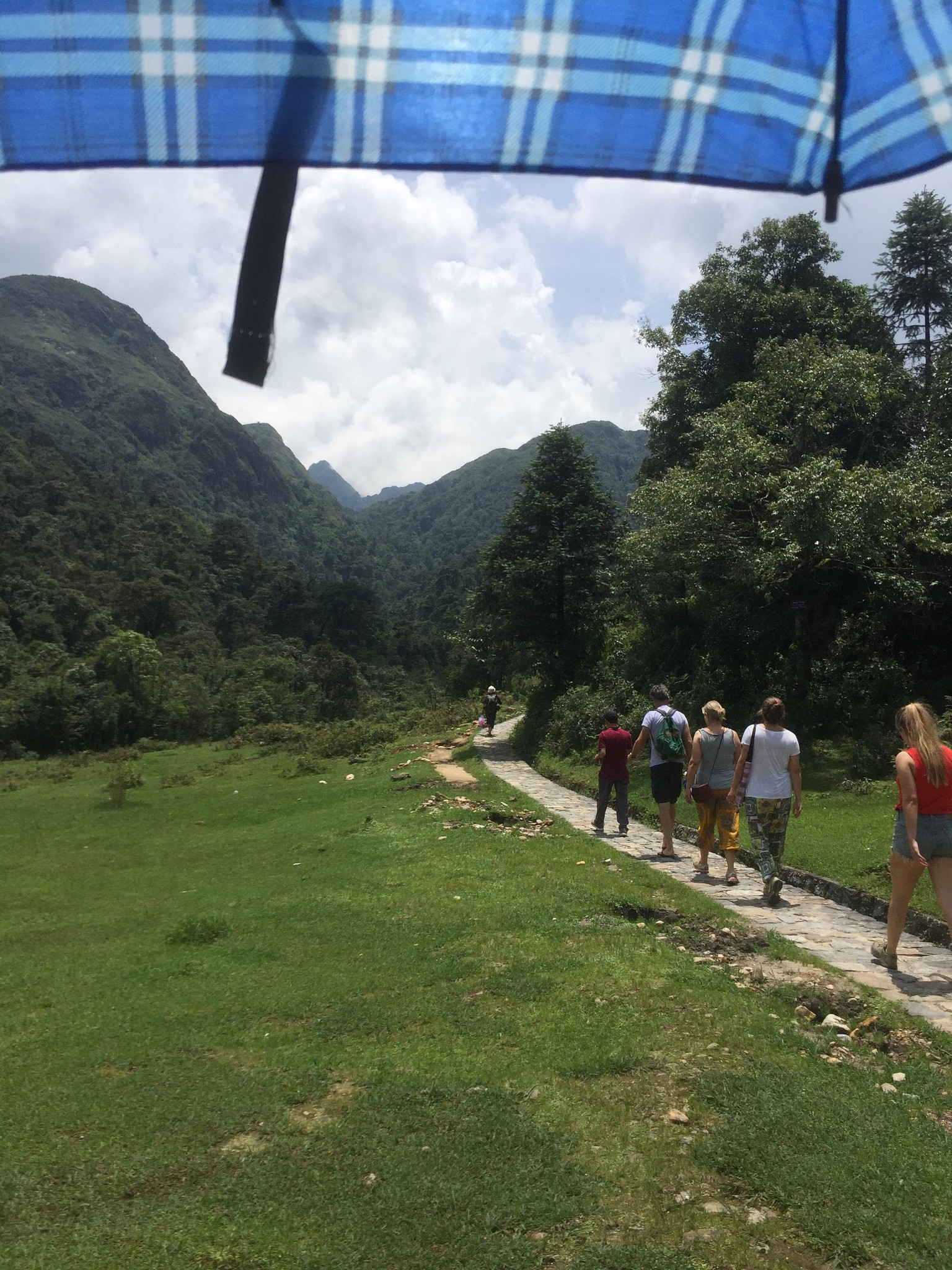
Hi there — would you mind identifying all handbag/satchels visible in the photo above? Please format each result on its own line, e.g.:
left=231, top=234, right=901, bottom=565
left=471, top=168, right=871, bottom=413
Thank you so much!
left=692, top=783, right=714, bottom=801
left=732, top=723, right=757, bottom=807
left=479, top=715, right=485, bottom=729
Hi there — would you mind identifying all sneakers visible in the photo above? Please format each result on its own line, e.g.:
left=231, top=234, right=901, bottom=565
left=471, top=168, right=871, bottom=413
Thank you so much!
left=767, top=876, right=782, bottom=906
left=871, top=937, right=898, bottom=970
left=763, top=885, right=768, bottom=896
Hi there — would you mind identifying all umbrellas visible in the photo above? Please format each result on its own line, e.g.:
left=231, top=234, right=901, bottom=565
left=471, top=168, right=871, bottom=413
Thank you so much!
left=1, top=0, right=951, bottom=385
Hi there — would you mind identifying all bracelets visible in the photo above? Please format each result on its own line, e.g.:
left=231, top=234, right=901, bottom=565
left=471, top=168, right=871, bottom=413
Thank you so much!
left=630, top=753, right=636, bottom=758
left=795, top=799, right=801, bottom=802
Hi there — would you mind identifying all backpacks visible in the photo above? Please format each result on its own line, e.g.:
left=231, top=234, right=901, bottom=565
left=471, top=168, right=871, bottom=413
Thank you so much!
left=649, top=708, right=687, bottom=761
left=486, top=695, right=496, bottom=713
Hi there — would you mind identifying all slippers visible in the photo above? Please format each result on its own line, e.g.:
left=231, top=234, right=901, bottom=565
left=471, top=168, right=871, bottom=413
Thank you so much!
left=657, top=850, right=675, bottom=859
left=662, top=843, right=667, bottom=849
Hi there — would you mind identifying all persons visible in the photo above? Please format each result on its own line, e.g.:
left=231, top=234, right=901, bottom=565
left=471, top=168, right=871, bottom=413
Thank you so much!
left=627, top=684, right=693, bottom=858
left=481, top=685, right=502, bottom=736
left=871, top=702, right=952, bottom=970
left=728, top=697, right=802, bottom=907
left=591, top=710, right=633, bottom=836
left=685, top=700, right=742, bottom=883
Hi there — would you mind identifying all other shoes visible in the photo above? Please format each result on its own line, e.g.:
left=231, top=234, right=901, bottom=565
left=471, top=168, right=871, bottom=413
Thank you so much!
left=619, top=828, right=626, bottom=836
left=726, top=871, right=739, bottom=883
left=693, top=861, right=709, bottom=873
left=487, top=729, right=493, bottom=736
left=591, top=818, right=604, bottom=831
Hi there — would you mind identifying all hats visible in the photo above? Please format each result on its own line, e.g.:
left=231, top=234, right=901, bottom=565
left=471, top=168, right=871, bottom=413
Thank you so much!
left=487, top=686, right=496, bottom=693
